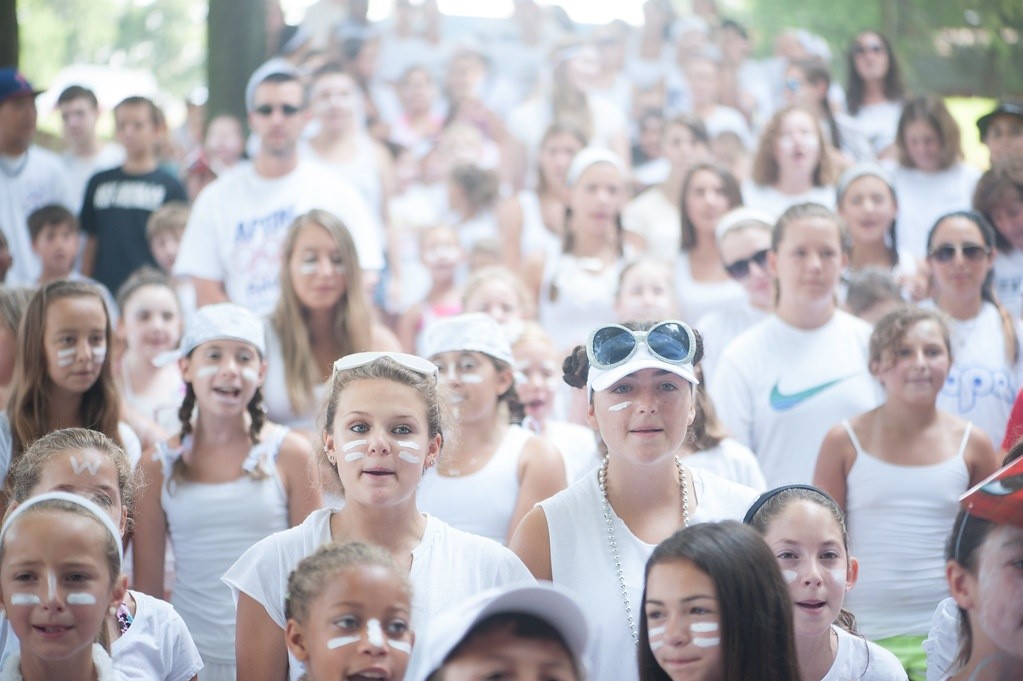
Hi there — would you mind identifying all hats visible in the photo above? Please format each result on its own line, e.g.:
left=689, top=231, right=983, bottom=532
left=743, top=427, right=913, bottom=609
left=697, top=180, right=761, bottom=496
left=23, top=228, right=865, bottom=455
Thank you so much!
left=0, top=67, right=45, bottom=105
left=703, top=107, right=752, bottom=149
left=583, top=337, right=699, bottom=394
left=977, top=102, right=1023, bottom=139
left=244, top=54, right=304, bottom=95
left=709, top=206, right=781, bottom=256
left=565, top=147, right=626, bottom=185
left=150, top=304, right=268, bottom=368
left=413, top=314, right=516, bottom=369
left=415, top=581, right=590, bottom=681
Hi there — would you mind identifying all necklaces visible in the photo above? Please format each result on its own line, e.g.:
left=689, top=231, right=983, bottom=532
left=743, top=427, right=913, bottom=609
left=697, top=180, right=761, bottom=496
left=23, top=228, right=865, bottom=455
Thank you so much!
left=599, top=452, right=691, bottom=649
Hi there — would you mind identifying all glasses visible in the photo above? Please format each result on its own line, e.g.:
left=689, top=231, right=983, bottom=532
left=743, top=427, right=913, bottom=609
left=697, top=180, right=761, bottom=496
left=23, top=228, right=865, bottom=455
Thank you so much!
left=330, top=351, right=440, bottom=387
left=727, top=251, right=775, bottom=282
left=250, top=104, right=300, bottom=116
left=586, top=320, right=695, bottom=365
left=925, top=244, right=991, bottom=262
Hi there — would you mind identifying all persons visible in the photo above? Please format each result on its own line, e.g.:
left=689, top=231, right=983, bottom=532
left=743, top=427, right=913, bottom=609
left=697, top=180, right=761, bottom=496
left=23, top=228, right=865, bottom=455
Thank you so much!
left=115, top=262, right=191, bottom=436
left=412, top=312, right=570, bottom=548
left=742, top=484, right=909, bottom=681
left=415, top=582, right=590, bottom=681
left=810, top=308, right=999, bottom=681
left=940, top=454, right=1023, bottom=681
left=637, top=519, right=804, bottom=681
left=0, top=0, right=1023, bottom=302
left=511, top=322, right=750, bottom=681
left=706, top=201, right=886, bottom=493
left=0, top=490, right=134, bottom=681
left=256, top=212, right=404, bottom=516
left=0, top=425, right=204, bottom=681
left=131, top=303, right=325, bottom=681
left=283, top=541, right=416, bottom=681
left=221, top=351, right=537, bottom=681
left=912, top=212, right=1023, bottom=455
left=0, top=281, right=141, bottom=526
